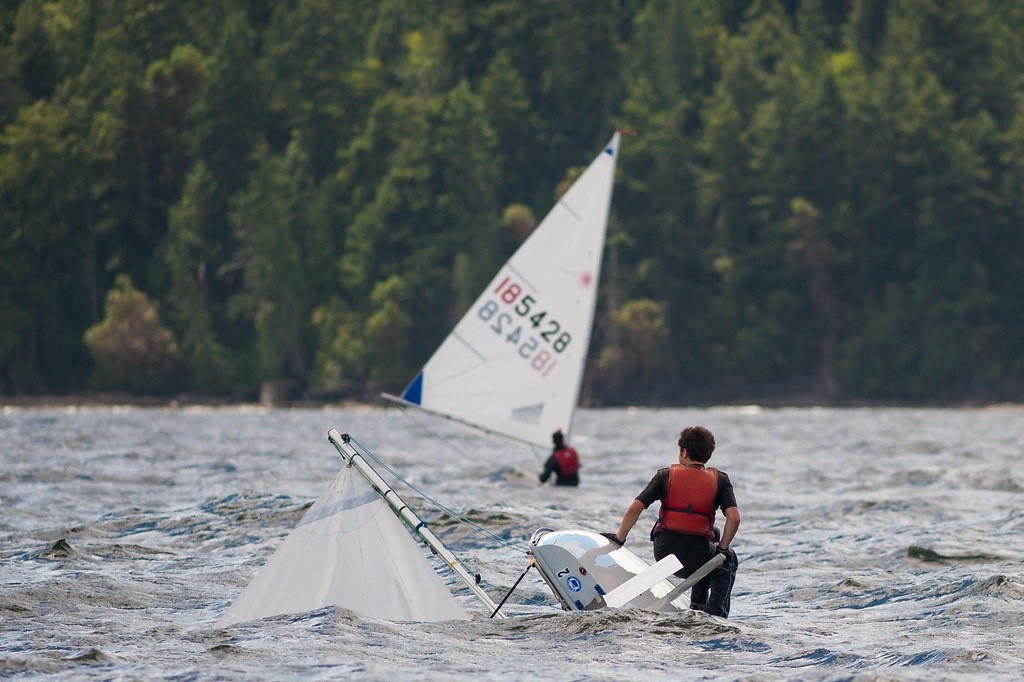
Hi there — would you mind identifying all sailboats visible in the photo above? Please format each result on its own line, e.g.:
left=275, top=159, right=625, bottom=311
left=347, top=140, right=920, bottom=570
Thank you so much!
left=376, top=126, right=635, bottom=492
left=206, top=424, right=730, bottom=626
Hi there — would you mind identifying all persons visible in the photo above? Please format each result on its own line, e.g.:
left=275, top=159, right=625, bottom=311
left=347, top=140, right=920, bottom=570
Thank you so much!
left=540, top=432, right=580, bottom=487
left=600, top=426, right=741, bottom=619
